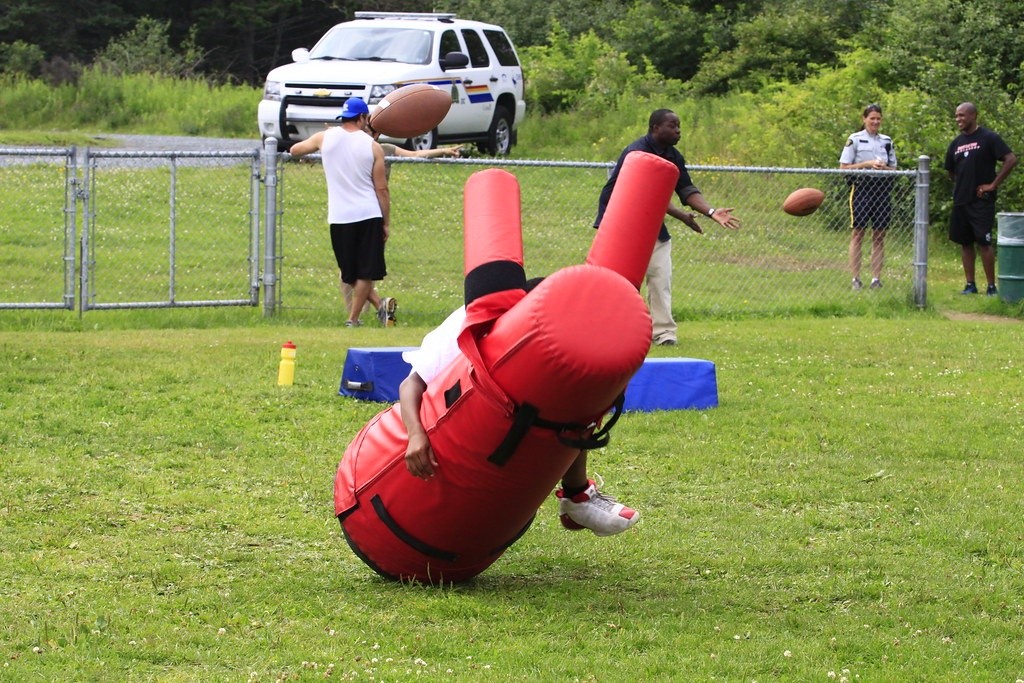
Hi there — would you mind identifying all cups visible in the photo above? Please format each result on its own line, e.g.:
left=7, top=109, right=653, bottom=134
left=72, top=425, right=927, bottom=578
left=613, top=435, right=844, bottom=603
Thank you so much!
left=278, top=340, right=296, bottom=385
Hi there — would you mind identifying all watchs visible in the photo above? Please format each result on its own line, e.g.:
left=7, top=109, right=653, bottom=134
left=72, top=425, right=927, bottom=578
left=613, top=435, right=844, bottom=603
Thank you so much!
left=705, top=207, right=715, bottom=218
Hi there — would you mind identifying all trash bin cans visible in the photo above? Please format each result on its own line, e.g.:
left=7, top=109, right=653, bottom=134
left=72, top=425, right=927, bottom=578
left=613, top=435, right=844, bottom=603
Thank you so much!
left=994, top=212, right=1024, bottom=303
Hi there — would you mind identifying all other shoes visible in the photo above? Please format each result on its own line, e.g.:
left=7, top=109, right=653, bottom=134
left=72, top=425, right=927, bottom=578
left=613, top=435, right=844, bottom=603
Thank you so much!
left=852, top=278, right=863, bottom=290
left=870, top=279, right=881, bottom=288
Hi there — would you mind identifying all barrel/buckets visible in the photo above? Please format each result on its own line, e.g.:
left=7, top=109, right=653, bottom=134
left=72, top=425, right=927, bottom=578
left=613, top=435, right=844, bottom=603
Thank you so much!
left=997, top=210, right=1024, bottom=306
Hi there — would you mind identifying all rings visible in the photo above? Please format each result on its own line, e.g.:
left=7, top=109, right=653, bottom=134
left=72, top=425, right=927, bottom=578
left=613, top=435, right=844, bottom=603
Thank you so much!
left=979, top=189, right=982, bottom=191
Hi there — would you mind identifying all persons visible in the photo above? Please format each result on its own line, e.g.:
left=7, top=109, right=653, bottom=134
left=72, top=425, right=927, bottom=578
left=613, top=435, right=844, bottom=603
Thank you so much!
left=290, top=97, right=397, bottom=327
left=399, top=276, right=642, bottom=539
left=323, top=114, right=464, bottom=328
left=837, top=105, right=898, bottom=289
left=590, top=107, right=740, bottom=347
left=944, top=102, right=1018, bottom=296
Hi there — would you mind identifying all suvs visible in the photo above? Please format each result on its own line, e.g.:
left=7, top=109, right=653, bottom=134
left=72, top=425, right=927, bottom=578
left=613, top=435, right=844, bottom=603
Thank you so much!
left=256, top=12, right=526, bottom=151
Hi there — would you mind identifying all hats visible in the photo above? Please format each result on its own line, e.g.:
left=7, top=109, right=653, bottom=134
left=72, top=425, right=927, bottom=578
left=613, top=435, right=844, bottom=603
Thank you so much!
left=335, top=96, right=369, bottom=124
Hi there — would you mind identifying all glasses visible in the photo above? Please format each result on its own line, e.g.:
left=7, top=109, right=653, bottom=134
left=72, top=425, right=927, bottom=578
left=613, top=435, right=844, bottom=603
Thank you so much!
left=866, top=103, right=882, bottom=112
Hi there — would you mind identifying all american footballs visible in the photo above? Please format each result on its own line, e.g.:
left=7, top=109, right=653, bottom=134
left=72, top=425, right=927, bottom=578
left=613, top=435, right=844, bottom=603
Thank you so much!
left=368, top=85, right=452, bottom=138
left=782, top=187, right=826, bottom=216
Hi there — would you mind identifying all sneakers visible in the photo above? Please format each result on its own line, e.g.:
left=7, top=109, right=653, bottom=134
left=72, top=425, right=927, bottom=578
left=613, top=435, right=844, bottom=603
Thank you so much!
left=960, top=282, right=977, bottom=294
left=374, top=293, right=396, bottom=329
left=557, top=482, right=640, bottom=537
left=986, top=284, right=998, bottom=297
left=343, top=319, right=365, bottom=328
